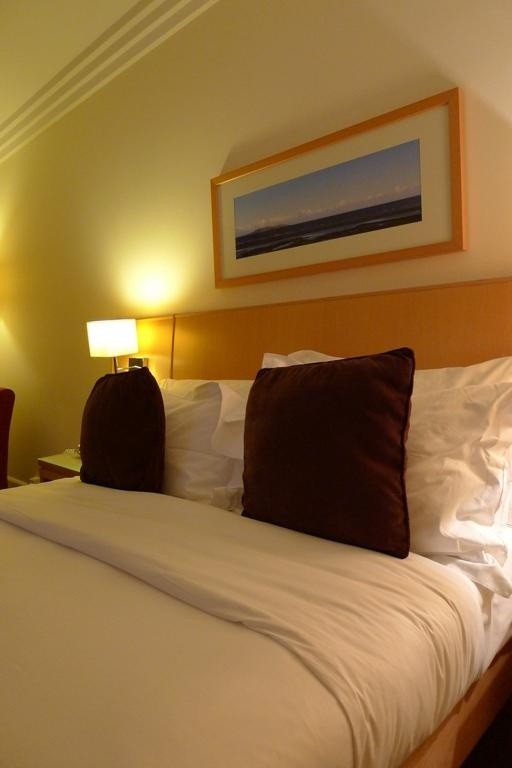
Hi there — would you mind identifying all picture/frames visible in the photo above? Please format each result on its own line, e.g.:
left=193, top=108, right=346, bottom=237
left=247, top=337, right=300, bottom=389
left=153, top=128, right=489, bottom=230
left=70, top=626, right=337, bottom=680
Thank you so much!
left=209, top=83, right=468, bottom=289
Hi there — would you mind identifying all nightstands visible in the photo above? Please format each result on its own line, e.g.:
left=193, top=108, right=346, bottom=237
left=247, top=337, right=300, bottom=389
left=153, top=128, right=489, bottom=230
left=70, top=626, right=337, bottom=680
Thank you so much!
left=36, top=448, right=80, bottom=483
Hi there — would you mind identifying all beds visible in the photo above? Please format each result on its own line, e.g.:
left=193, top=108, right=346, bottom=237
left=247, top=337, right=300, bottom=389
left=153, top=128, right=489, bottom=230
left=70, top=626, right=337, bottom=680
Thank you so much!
left=1, top=278, right=512, bottom=767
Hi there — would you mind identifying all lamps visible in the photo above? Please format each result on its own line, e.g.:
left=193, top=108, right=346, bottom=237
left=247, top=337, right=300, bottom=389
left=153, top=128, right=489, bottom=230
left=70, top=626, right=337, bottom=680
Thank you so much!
left=84, top=317, right=147, bottom=374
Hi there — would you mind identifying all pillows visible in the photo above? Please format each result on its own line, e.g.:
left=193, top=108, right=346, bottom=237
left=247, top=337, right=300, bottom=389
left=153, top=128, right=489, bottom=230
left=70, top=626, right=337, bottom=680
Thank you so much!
left=153, top=376, right=256, bottom=510
left=79, top=366, right=165, bottom=493
left=260, top=350, right=512, bottom=598
left=241, top=346, right=416, bottom=561
